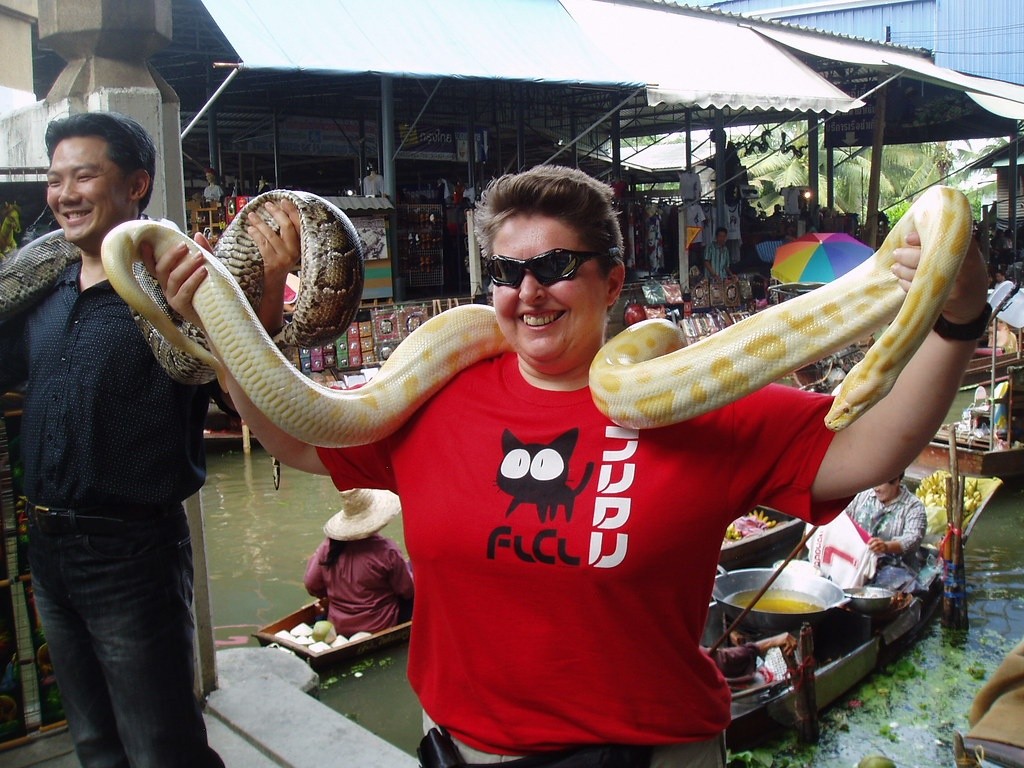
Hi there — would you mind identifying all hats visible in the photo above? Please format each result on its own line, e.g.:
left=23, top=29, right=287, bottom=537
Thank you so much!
left=323, top=487, right=402, bottom=542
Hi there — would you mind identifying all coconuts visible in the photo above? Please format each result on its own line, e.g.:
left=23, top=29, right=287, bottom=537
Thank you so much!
left=273, top=620, right=374, bottom=653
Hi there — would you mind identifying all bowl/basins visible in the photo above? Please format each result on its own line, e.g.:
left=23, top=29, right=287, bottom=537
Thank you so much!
left=841, top=587, right=893, bottom=618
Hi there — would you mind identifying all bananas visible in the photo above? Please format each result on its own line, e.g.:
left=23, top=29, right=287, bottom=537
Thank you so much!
left=914, top=471, right=982, bottom=529
left=724, top=523, right=743, bottom=540
left=745, top=510, right=776, bottom=528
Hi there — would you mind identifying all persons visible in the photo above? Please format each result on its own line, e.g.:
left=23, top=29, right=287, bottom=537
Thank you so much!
left=0, top=111, right=301, bottom=768
left=845, top=471, right=927, bottom=591
left=995, top=269, right=1008, bottom=289
left=204, top=168, right=224, bottom=202
left=305, top=486, right=413, bottom=637
left=988, top=318, right=1018, bottom=355
left=363, top=170, right=384, bottom=196
left=704, top=227, right=730, bottom=284
left=141, top=166, right=990, bottom=768
left=700, top=632, right=797, bottom=689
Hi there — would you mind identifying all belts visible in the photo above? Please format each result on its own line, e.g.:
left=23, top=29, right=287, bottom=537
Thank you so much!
left=13, top=496, right=152, bottom=534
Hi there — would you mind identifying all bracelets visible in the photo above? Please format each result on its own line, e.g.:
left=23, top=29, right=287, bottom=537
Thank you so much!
left=932, top=303, right=994, bottom=341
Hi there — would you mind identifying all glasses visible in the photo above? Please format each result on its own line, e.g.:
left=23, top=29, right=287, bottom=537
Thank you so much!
left=484, top=246, right=620, bottom=289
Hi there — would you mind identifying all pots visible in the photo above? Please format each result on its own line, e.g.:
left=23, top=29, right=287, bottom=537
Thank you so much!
left=710, top=564, right=851, bottom=628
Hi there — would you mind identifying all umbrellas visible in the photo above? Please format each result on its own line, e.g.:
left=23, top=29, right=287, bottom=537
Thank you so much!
left=771, top=233, right=874, bottom=285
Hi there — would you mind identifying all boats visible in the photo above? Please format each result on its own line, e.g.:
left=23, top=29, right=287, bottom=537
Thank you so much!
left=249, top=595, right=414, bottom=673
left=705, top=343, right=1023, bottom=745
